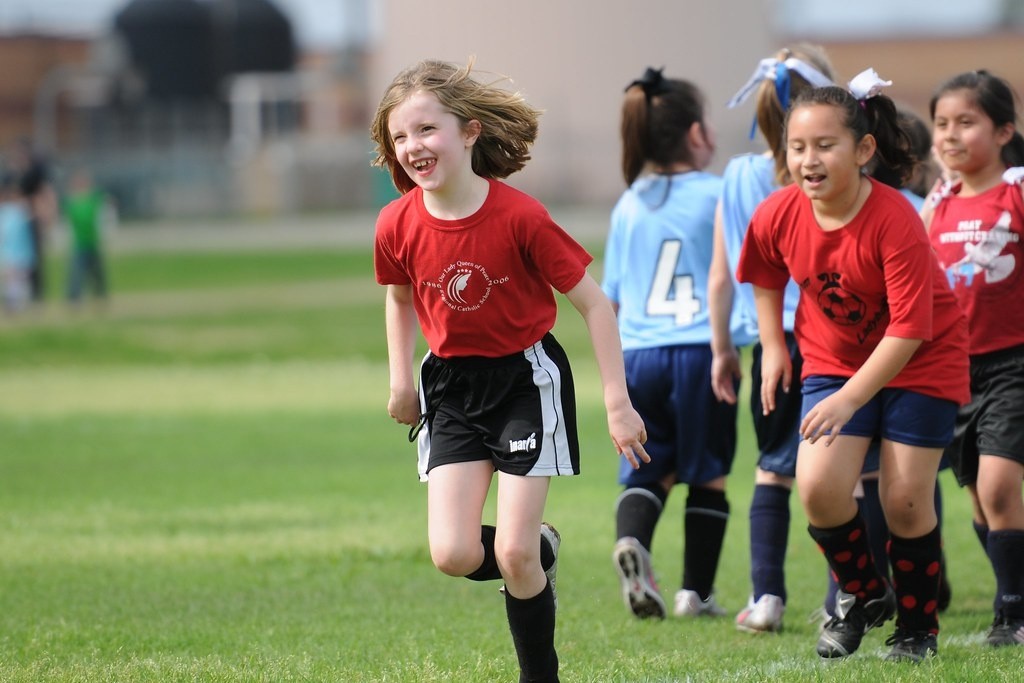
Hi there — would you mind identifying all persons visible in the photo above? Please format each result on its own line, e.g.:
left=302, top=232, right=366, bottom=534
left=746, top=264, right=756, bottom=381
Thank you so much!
left=13, top=141, right=106, bottom=303
left=708, top=41, right=843, bottom=633
left=736, top=68, right=971, bottom=666
left=602, top=64, right=752, bottom=621
left=919, top=68, right=1024, bottom=646
left=369, top=57, right=651, bottom=683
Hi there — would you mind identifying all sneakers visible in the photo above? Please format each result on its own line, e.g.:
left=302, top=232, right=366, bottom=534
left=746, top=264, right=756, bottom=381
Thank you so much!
left=882, top=628, right=937, bottom=665
left=674, top=589, right=728, bottom=618
left=816, top=585, right=894, bottom=660
left=613, top=537, right=667, bottom=622
left=734, top=593, right=784, bottom=633
left=985, top=613, right=1024, bottom=645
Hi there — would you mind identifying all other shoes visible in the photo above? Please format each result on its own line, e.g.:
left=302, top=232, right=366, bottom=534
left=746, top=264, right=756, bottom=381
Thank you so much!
left=499, top=523, right=561, bottom=610
